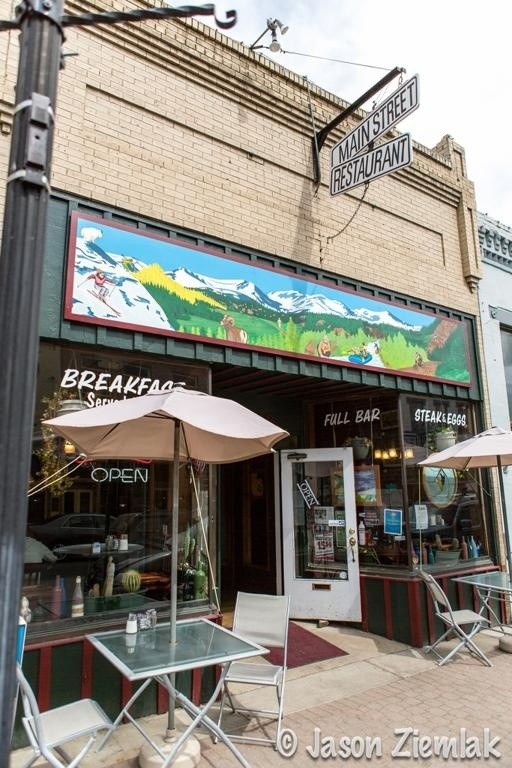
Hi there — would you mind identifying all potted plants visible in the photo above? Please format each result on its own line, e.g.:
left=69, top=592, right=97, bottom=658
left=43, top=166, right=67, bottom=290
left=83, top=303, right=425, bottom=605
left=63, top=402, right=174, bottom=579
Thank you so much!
left=83, top=558, right=148, bottom=611
left=430, top=424, right=459, bottom=452
left=342, top=435, right=374, bottom=459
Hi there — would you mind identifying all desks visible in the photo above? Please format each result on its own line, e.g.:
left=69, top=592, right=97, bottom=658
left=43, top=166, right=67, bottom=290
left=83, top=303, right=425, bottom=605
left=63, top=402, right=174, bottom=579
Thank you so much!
left=85, top=615, right=270, bottom=768
left=450, top=570, right=512, bottom=645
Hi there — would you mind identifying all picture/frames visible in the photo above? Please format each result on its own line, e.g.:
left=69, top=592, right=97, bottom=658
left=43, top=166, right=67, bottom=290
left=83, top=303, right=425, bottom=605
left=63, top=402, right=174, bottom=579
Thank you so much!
left=422, top=453, right=459, bottom=509
left=325, top=464, right=381, bottom=509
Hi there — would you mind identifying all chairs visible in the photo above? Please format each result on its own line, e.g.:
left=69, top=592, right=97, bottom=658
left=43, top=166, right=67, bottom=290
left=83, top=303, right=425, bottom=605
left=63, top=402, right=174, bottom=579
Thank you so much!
left=19, top=561, right=52, bottom=620
left=413, top=568, right=486, bottom=670
left=16, top=661, right=103, bottom=768
left=221, top=586, right=293, bottom=751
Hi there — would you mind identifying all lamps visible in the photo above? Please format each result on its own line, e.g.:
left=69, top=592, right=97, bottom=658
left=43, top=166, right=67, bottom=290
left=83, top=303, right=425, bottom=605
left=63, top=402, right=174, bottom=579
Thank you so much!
left=253, top=17, right=288, bottom=59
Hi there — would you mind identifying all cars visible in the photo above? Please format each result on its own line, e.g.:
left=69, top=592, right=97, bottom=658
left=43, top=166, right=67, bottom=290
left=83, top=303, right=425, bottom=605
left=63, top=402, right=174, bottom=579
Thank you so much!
left=310, top=470, right=488, bottom=564
left=19, top=501, right=213, bottom=640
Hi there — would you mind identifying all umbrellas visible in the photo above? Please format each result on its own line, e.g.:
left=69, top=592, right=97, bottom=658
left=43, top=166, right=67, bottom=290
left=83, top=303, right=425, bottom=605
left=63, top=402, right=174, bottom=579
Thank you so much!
left=44, top=380, right=291, bottom=738
left=417, top=424, right=512, bottom=583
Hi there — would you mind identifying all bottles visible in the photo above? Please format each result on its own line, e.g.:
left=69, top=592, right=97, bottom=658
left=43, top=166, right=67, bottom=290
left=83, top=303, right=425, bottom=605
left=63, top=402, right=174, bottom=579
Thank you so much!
left=50, top=574, right=66, bottom=618
left=71, top=576, right=86, bottom=618
left=125, top=612, right=138, bottom=636
left=138, top=608, right=157, bottom=630
left=460, top=535, right=478, bottom=560
left=106, top=533, right=129, bottom=551
left=415, top=543, right=434, bottom=564
left=358, top=519, right=368, bottom=546
left=125, top=631, right=158, bottom=656
left=195, top=561, right=206, bottom=600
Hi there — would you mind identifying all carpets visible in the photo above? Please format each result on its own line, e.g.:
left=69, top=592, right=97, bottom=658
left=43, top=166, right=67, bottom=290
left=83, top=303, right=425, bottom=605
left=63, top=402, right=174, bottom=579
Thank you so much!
left=228, top=619, right=350, bottom=671
